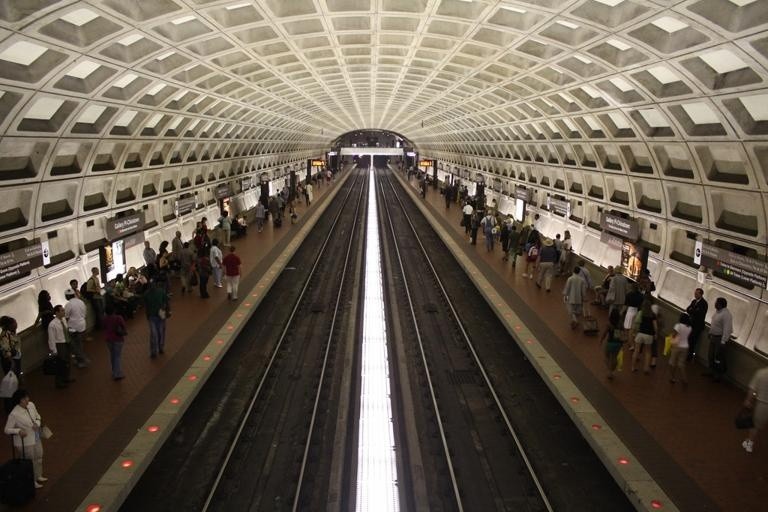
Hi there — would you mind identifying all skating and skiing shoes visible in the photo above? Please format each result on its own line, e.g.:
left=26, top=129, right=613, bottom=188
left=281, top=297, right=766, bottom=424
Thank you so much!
left=150, top=351, right=166, bottom=360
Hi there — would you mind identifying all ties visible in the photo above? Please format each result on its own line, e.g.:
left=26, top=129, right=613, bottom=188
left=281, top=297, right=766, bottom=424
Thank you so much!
left=61, top=321, right=70, bottom=346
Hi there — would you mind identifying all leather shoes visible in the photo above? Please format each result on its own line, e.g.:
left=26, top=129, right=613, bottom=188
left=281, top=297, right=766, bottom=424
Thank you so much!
left=37, top=475, right=49, bottom=482
left=34, top=480, right=44, bottom=488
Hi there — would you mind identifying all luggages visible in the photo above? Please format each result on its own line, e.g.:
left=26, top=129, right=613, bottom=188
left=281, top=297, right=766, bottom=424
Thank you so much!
left=1, top=426, right=35, bottom=508
left=581, top=299, right=600, bottom=336
left=290, top=213, right=298, bottom=224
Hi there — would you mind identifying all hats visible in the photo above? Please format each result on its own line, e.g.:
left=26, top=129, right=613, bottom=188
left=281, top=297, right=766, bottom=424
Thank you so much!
left=542, top=238, right=554, bottom=247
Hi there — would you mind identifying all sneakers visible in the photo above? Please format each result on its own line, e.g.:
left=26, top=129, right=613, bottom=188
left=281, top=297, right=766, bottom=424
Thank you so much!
left=535, top=281, right=551, bottom=293
left=258, top=226, right=265, bottom=233
left=631, top=364, right=657, bottom=375
left=180, top=284, right=194, bottom=296
left=111, top=374, right=126, bottom=381
left=213, top=282, right=241, bottom=303
left=669, top=376, right=690, bottom=385
left=742, top=439, right=755, bottom=456
left=521, top=271, right=534, bottom=280
left=222, top=242, right=232, bottom=247
left=570, top=320, right=580, bottom=330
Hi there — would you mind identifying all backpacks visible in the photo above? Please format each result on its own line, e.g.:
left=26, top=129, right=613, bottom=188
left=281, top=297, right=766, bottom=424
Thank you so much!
left=80, top=277, right=96, bottom=301
left=527, top=245, right=539, bottom=261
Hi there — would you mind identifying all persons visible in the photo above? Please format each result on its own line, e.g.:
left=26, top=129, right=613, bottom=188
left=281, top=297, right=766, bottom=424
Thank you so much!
left=742, top=367, right=768, bottom=453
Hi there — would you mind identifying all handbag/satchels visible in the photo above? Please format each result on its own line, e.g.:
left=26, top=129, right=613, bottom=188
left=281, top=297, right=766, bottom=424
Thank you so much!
left=0, top=369, right=20, bottom=399
left=490, top=225, right=501, bottom=234
left=189, top=272, right=198, bottom=286
left=158, top=286, right=167, bottom=321
left=273, top=213, right=282, bottom=228
left=34, top=417, right=54, bottom=440
left=615, top=344, right=625, bottom=373
left=734, top=403, right=756, bottom=430
left=42, top=356, right=57, bottom=376
left=301, top=185, right=307, bottom=195
left=6, top=331, right=17, bottom=357
left=112, top=316, right=128, bottom=336
left=194, top=235, right=202, bottom=242
left=662, top=333, right=674, bottom=357
